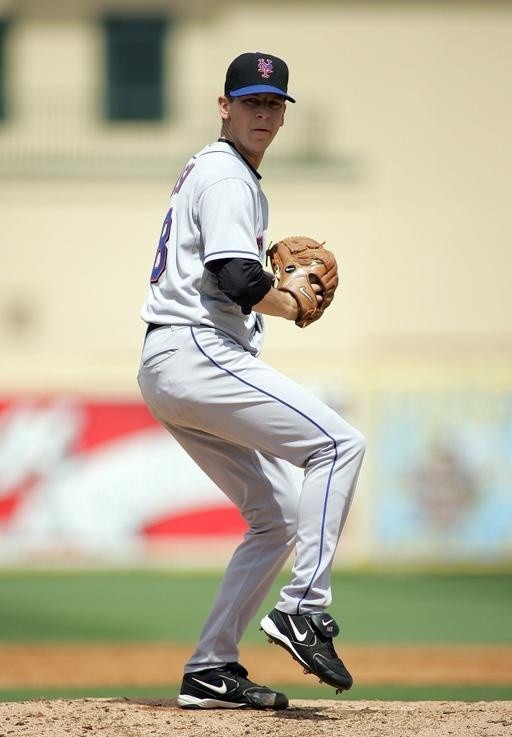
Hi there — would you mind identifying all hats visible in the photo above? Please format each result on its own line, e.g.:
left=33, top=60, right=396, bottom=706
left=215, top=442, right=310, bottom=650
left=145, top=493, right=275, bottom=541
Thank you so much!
left=227, top=52, right=295, bottom=103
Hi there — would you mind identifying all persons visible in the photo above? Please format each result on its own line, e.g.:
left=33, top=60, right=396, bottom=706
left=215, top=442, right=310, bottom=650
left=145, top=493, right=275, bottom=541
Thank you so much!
left=139, top=53, right=366, bottom=709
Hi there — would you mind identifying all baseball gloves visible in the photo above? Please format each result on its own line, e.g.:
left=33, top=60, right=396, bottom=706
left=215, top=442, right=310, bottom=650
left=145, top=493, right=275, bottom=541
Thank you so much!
left=265, top=237, right=339, bottom=327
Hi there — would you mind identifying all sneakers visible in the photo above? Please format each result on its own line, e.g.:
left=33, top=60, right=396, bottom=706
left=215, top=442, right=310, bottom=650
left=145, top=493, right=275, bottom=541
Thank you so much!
left=260, top=607, right=353, bottom=690
left=178, top=660, right=289, bottom=709
